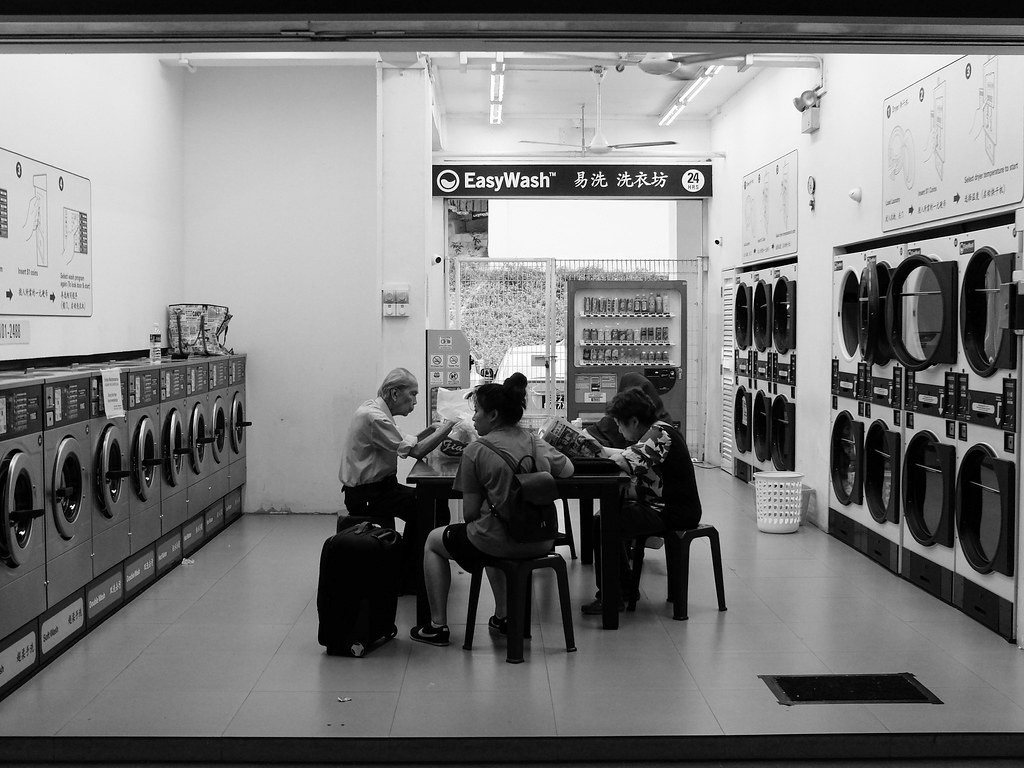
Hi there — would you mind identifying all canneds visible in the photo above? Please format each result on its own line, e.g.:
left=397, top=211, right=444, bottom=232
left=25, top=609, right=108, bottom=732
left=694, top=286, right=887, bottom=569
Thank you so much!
left=584, top=297, right=669, bottom=364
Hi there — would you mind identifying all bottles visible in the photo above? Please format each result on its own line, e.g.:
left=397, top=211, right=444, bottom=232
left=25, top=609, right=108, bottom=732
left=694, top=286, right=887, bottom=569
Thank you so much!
left=648, top=292, right=655, bottom=314
left=640, top=294, right=648, bottom=314
left=663, top=292, right=670, bottom=313
left=149, top=322, right=162, bottom=364
left=634, top=294, right=641, bottom=314
left=654, top=292, right=663, bottom=314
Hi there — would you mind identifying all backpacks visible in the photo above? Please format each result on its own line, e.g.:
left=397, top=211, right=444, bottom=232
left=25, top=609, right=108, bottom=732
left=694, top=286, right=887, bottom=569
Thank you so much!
left=476, top=437, right=558, bottom=542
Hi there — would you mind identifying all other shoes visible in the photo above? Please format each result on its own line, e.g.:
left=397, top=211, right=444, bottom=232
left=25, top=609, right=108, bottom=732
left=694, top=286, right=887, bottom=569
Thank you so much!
left=582, top=595, right=627, bottom=615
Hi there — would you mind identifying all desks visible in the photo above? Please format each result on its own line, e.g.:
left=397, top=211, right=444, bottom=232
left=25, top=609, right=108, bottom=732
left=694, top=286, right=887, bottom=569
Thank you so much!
left=406, top=442, right=632, bottom=630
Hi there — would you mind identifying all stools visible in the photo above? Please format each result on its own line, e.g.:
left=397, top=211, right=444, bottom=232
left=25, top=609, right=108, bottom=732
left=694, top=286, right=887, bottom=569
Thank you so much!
left=336, top=509, right=402, bottom=597
left=462, top=550, right=577, bottom=664
left=552, top=498, right=577, bottom=560
left=626, top=524, right=727, bottom=620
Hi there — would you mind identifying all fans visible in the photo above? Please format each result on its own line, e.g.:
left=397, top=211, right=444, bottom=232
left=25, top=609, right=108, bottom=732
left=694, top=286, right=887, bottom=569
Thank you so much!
left=519, top=83, right=676, bottom=153
left=560, top=51, right=745, bottom=80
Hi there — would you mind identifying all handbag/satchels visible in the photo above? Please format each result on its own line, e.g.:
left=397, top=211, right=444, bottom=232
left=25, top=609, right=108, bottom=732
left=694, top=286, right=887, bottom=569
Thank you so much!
left=436, top=386, right=479, bottom=462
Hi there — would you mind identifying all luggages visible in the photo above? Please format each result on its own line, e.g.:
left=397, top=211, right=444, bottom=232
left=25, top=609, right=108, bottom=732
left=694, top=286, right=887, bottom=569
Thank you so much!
left=317, top=519, right=402, bottom=658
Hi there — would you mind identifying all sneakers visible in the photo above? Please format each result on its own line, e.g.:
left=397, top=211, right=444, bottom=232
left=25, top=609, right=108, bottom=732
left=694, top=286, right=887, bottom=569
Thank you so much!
left=409, top=621, right=451, bottom=647
left=488, top=615, right=510, bottom=636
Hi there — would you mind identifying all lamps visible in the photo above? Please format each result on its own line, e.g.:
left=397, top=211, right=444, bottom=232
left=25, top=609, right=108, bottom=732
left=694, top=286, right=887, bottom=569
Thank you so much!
left=658, top=65, right=724, bottom=126
left=794, top=90, right=820, bottom=112
left=489, top=63, right=505, bottom=124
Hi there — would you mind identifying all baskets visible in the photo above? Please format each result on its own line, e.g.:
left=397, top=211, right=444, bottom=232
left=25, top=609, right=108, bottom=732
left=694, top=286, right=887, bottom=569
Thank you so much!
left=754, top=470, right=803, bottom=534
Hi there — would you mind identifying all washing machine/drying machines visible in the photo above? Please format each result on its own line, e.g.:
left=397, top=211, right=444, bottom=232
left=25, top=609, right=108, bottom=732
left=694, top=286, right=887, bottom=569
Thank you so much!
left=733, top=253, right=798, bottom=473
left=0, top=352, right=247, bottom=704
left=828, top=207, right=1024, bottom=603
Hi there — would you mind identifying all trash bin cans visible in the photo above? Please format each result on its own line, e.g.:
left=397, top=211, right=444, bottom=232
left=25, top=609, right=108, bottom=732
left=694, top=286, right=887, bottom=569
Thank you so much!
left=753, top=471, right=804, bottom=534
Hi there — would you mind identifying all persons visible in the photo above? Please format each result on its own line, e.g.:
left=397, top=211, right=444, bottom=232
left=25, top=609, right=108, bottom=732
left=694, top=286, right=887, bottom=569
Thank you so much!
left=579, top=388, right=702, bottom=615
left=337, top=367, right=451, bottom=595
left=586, top=371, right=672, bottom=550
left=408, top=372, right=575, bottom=644
